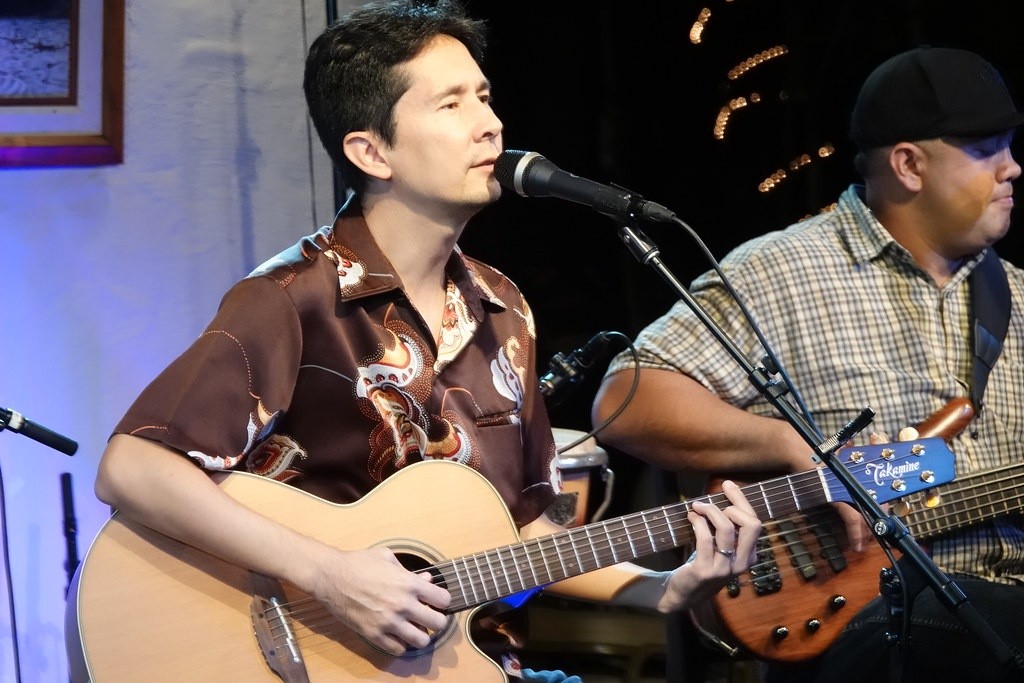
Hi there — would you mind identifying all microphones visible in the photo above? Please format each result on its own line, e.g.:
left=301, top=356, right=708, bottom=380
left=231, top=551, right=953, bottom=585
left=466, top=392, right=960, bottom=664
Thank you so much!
left=493, top=149, right=675, bottom=223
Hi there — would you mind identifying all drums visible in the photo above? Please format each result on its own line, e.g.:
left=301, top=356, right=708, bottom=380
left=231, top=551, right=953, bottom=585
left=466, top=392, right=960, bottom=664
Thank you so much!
left=547, top=427, right=596, bottom=529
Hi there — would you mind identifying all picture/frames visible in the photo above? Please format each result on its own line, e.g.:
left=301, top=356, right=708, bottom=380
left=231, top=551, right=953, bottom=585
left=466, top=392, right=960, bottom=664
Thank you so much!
left=0, top=1, right=130, bottom=174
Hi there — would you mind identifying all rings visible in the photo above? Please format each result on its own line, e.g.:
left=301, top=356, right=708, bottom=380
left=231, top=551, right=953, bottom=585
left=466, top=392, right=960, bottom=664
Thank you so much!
left=715, top=546, right=736, bottom=555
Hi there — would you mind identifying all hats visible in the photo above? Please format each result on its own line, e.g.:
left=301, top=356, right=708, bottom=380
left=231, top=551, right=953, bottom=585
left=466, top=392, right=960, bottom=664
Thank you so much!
left=849, top=48, right=1020, bottom=151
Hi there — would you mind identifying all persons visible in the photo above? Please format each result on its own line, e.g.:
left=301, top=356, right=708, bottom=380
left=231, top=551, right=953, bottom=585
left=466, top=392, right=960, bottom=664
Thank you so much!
left=587, top=51, right=1023, bottom=683
left=92, top=0, right=761, bottom=682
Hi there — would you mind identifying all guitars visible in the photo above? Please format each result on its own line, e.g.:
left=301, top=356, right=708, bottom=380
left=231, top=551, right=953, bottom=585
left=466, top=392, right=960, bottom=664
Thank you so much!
left=684, top=457, right=1023, bottom=661
left=61, top=436, right=960, bottom=683
